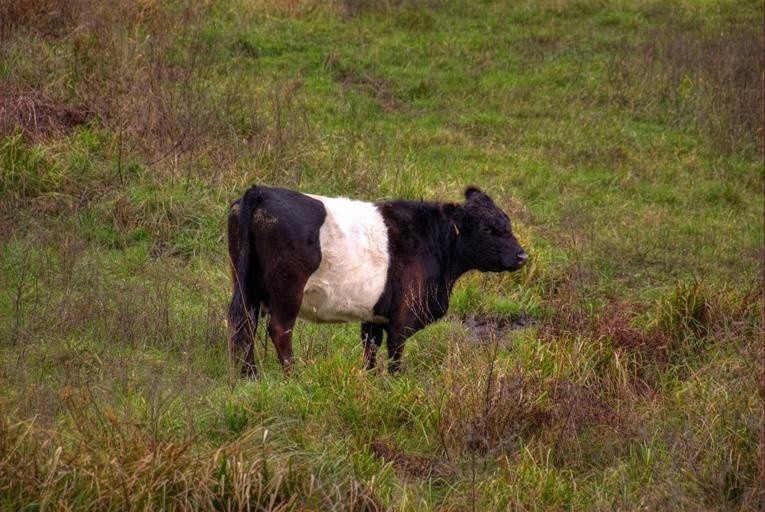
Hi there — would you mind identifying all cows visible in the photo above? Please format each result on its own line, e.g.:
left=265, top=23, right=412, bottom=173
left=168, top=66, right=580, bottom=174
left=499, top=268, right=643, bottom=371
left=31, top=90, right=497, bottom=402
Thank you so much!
left=223, top=184, right=530, bottom=379
